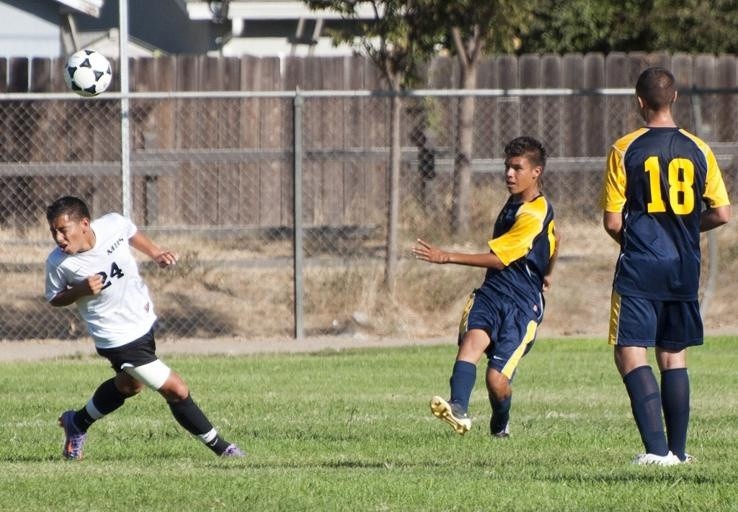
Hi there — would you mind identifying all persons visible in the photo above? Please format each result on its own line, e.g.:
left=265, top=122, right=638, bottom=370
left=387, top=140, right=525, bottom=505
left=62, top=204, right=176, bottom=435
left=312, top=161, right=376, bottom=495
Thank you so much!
left=411, top=134, right=561, bottom=438
left=41, top=193, right=243, bottom=460
left=602, top=66, right=733, bottom=467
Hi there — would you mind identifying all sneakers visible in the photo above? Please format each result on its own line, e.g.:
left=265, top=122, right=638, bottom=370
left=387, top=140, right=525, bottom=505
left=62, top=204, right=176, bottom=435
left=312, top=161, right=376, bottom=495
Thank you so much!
left=490, top=412, right=510, bottom=438
left=633, top=451, right=698, bottom=465
left=220, top=443, right=244, bottom=458
left=58, top=410, right=84, bottom=461
left=430, top=396, right=471, bottom=436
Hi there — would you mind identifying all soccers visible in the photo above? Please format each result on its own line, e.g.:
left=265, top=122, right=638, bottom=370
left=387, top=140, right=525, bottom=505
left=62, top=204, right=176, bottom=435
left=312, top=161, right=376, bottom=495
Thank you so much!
left=63, top=48, right=114, bottom=96
